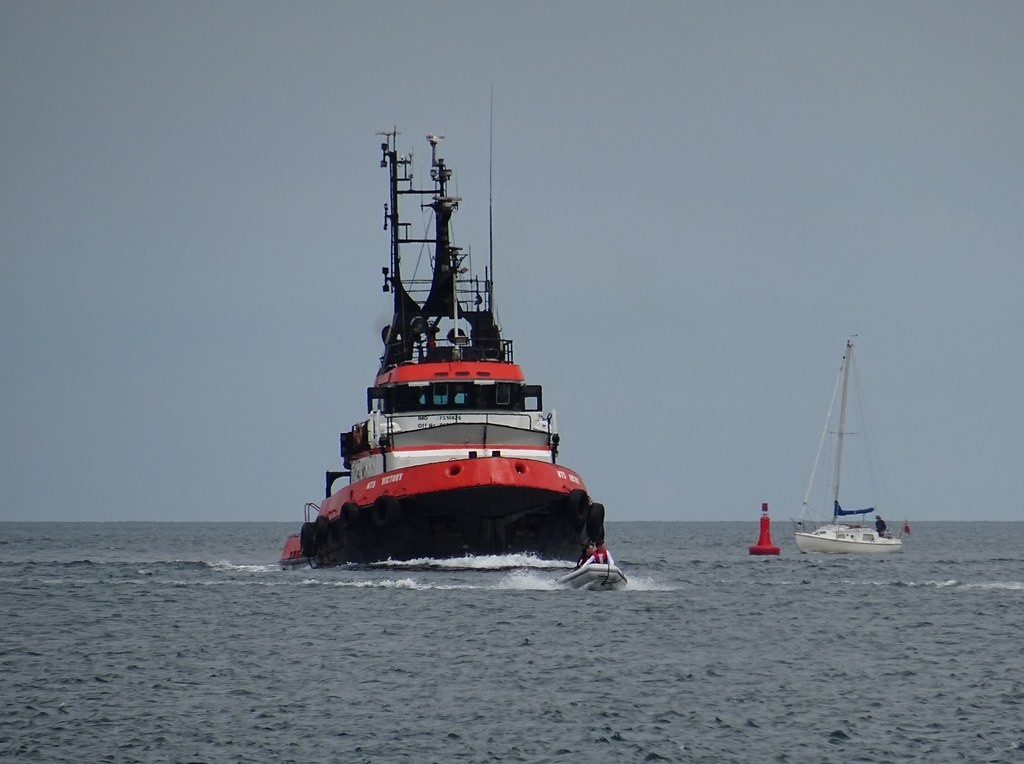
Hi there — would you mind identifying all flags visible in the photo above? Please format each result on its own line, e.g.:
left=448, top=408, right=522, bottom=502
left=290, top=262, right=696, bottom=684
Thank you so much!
left=904, top=519, right=910, bottom=535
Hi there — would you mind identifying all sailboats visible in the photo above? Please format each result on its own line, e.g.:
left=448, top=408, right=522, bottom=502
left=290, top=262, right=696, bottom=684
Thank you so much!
left=789, top=339, right=910, bottom=552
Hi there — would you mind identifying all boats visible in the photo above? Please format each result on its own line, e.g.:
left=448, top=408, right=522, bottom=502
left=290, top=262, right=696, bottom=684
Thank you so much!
left=278, top=83, right=608, bottom=572
left=556, top=562, right=629, bottom=591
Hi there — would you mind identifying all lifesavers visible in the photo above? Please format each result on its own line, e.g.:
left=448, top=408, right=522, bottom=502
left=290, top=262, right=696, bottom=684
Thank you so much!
left=352, top=422, right=363, bottom=445
